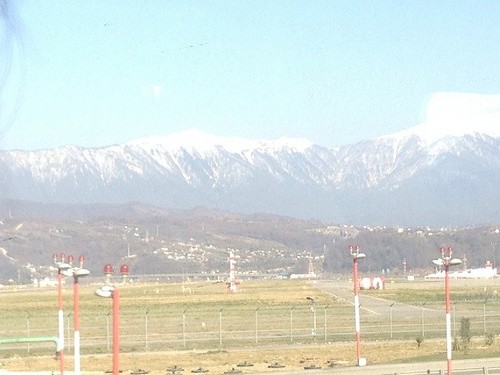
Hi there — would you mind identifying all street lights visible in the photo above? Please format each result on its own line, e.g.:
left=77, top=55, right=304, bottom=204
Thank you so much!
left=349, top=246, right=366, bottom=368
left=52, top=254, right=129, bottom=375
left=432, top=247, right=463, bottom=375
